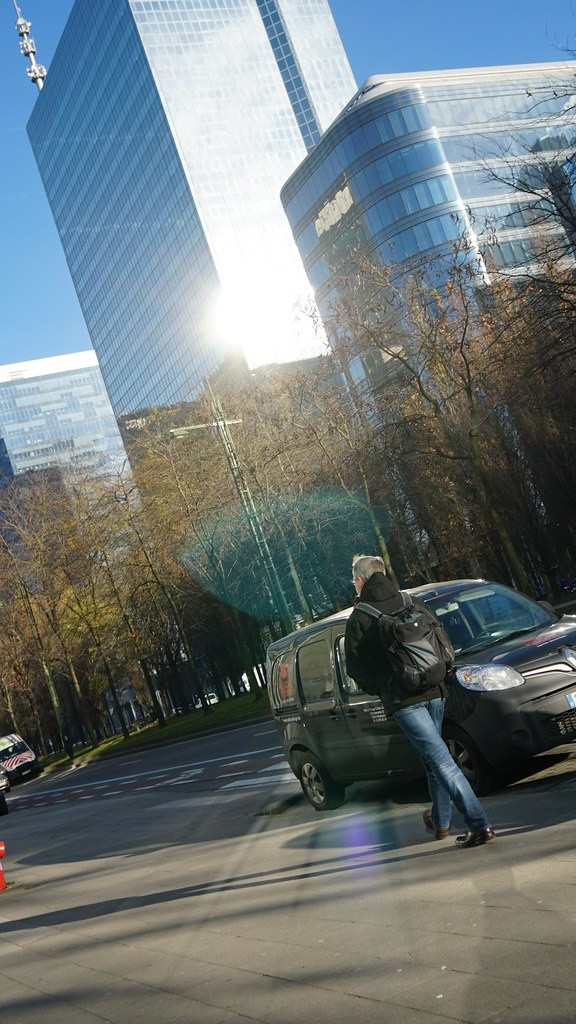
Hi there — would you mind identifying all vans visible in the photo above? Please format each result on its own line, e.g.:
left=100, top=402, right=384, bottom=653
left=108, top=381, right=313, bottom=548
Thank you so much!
left=193, top=693, right=219, bottom=710
left=0, top=732, right=41, bottom=784
left=265, top=579, right=575, bottom=811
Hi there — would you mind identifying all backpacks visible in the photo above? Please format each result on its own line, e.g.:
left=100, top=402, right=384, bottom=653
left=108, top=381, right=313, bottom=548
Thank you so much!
left=356, top=591, right=448, bottom=704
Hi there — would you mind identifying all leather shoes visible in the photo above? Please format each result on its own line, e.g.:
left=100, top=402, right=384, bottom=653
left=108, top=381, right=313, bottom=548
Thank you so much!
left=456, top=827, right=496, bottom=847
left=424, top=808, right=448, bottom=840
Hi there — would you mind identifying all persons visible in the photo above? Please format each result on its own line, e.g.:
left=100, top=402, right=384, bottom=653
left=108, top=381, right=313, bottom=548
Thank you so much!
left=344, top=554, right=497, bottom=848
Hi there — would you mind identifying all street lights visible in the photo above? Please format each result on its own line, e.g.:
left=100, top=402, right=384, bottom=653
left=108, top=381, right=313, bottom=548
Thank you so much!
left=166, top=375, right=297, bottom=632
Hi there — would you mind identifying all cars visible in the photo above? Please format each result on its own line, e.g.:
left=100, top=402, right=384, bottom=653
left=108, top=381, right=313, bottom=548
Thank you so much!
left=0, top=775, right=12, bottom=794
